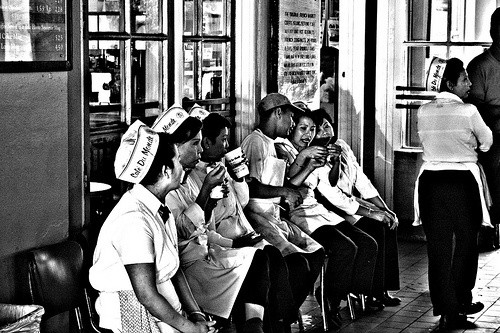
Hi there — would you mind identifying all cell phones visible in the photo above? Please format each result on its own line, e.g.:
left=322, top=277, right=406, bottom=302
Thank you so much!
left=324, top=135, right=338, bottom=147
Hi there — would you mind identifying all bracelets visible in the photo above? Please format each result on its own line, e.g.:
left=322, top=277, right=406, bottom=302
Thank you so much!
left=294, top=160, right=303, bottom=167
left=190, top=311, right=206, bottom=318
left=367, top=209, right=374, bottom=217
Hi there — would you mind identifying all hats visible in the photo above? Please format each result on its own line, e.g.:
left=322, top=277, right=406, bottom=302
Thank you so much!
left=256, top=92, right=310, bottom=113
left=423, top=56, right=447, bottom=93
left=152, top=103, right=211, bottom=135
left=113, top=119, right=160, bottom=185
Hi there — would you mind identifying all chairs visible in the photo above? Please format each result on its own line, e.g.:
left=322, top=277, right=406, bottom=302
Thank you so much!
left=26, top=218, right=368, bottom=333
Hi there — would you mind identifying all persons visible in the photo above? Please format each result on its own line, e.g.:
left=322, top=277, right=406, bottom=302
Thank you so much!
left=88, top=120, right=219, bottom=333
left=274, top=102, right=378, bottom=329
left=188, top=103, right=291, bottom=333
left=151, top=103, right=269, bottom=333
left=309, top=108, right=401, bottom=313
left=411, top=56, right=494, bottom=331
left=462, top=7, right=500, bottom=252
left=241, top=93, right=326, bottom=333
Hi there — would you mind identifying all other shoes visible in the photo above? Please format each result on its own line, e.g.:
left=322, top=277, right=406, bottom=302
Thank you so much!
left=439, top=306, right=476, bottom=331
left=263, top=313, right=282, bottom=333
left=236, top=315, right=265, bottom=333
left=373, top=291, right=402, bottom=306
left=459, top=301, right=484, bottom=315
left=353, top=305, right=378, bottom=318
left=315, top=286, right=343, bottom=330
left=280, top=318, right=292, bottom=333
left=364, top=300, right=384, bottom=312
